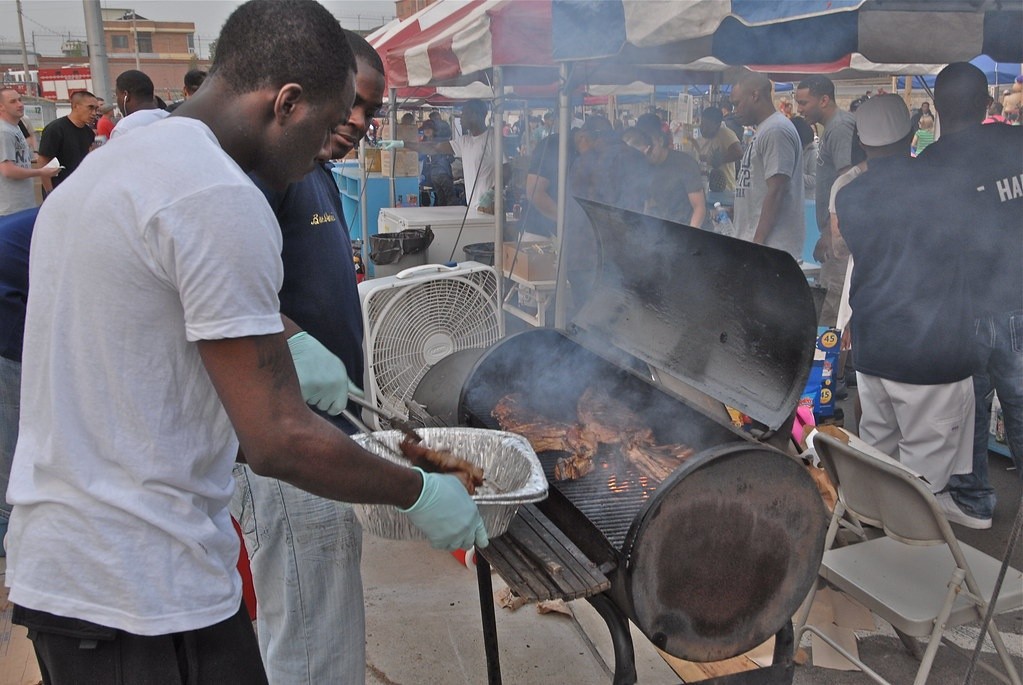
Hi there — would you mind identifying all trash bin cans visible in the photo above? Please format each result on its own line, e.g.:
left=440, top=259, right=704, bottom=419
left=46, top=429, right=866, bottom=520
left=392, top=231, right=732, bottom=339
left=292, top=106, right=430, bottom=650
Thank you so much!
left=463, top=242, right=518, bottom=307
left=369, top=232, right=429, bottom=277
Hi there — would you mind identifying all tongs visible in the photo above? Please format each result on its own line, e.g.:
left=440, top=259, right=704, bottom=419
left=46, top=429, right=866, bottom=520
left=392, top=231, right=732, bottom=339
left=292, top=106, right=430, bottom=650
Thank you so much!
left=341, top=392, right=421, bottom=456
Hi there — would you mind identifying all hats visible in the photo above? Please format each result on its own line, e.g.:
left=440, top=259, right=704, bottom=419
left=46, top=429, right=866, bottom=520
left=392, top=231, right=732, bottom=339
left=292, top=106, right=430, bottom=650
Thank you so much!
left=417, top=119, right=438, bottom=131
left=855, top=93, right=912, bottom=147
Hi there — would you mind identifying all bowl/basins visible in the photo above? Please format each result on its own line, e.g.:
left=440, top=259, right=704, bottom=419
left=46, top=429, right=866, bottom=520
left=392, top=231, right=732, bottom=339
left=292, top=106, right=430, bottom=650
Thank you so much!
left=344, top=426, right=549, bottom=541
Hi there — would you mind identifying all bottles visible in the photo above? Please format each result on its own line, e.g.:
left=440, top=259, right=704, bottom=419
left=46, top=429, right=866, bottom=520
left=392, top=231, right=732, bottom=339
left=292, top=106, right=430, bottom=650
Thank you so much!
left=714, top=202, right=736, bottom=237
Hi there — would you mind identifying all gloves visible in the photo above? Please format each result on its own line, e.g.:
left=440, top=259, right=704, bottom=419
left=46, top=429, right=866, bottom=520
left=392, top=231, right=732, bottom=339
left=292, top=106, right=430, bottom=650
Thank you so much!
left=395, top=468, right=488, bottom=550
left=286, top=332, right=364, bottom=416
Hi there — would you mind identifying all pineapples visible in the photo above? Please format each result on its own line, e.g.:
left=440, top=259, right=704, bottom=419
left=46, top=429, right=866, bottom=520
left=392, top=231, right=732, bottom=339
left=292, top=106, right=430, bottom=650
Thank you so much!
left=708, top=146, right=726, bottom=194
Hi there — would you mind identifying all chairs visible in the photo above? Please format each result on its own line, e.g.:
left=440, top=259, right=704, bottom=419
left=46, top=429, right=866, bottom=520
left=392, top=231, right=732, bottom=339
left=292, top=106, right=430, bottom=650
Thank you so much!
left=795, top=432, right=1023, bottom=685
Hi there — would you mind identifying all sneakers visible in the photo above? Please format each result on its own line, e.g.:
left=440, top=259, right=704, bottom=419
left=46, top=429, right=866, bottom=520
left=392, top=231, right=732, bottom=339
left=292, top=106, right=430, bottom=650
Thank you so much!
left=835, top=376, right=849, bottom=400
left=935, top=492, right=992, bottom=529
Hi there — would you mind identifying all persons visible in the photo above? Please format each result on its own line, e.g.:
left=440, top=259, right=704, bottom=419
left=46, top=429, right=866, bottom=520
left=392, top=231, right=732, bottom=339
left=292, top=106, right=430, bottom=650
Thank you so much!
left=247, top=26, right=384, bottom=683
left=3, top=0, right=472, bottom=685
left=0, top=70, right=208, bottom=555
left=375, top=63, right=1022, bottom=530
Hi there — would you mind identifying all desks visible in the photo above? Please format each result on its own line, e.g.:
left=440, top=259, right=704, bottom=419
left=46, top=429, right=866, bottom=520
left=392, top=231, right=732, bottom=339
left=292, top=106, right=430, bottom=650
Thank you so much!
left=491, top=266, right=571, bottom=326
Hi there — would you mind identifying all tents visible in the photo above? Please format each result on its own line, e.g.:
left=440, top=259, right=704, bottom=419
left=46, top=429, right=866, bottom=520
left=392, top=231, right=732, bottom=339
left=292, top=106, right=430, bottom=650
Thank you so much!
left=895, top=54, right=1023, bottom=89
left=364, top=0, right=549, bottom=337
left=363, top=17, right=654, bottom=137
left=612, top=81, right=793, bottom=104
left=739, top=53, right=948, bottom=75
left=550, top=0, right=1023, bottom=329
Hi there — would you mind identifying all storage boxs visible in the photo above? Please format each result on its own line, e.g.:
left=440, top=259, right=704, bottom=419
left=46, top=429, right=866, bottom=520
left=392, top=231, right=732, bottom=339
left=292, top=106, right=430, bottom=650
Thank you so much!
left=799, top=327, right=842, bottom=416
left=365, top=124, right=418, bottom=176
left=503, top=240, right=557, bottom=280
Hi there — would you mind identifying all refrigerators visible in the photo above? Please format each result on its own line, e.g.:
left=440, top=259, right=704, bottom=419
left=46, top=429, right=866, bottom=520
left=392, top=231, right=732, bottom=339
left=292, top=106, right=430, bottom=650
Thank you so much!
left=373, top=202, right=501, bottom=280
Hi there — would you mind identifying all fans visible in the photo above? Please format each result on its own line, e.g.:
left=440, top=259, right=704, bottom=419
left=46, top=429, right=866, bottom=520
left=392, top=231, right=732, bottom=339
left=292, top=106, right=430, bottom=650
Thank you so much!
left=357, top=261, right=502, bottom=431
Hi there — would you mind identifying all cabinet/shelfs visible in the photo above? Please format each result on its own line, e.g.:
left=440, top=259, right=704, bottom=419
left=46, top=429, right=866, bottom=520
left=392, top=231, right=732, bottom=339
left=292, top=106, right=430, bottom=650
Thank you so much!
left=331, top=167, right=419, bottom=276
